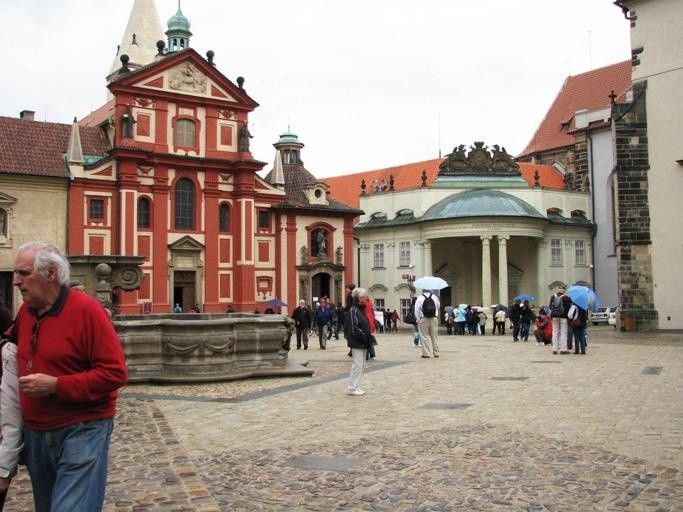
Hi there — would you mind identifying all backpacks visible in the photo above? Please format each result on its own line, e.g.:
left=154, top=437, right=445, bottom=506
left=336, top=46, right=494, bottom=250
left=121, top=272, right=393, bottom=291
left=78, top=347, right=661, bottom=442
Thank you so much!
left=550, top=295, right=564, bottom=316
left=419, top=294, right=435, bottom=317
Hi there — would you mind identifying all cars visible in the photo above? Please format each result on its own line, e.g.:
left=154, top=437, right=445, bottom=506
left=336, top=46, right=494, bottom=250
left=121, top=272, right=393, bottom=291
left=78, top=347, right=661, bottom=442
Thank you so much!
left=608, top=305, right=622, bottom=328
left=591, top=307, right=614, bottom=325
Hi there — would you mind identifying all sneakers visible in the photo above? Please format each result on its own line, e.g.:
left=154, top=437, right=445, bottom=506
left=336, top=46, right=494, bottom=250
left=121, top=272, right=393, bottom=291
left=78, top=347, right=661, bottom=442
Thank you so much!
left=348, top=391, right=363, bottom=395
left=535, top=340, right=587, bottom=355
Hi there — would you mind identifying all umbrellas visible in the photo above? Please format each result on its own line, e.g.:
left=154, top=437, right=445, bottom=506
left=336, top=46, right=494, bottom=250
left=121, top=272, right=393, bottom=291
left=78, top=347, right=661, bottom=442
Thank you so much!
left=412, top=276, right=449, bottom=291
left=566, top=285, right=602, bottom=313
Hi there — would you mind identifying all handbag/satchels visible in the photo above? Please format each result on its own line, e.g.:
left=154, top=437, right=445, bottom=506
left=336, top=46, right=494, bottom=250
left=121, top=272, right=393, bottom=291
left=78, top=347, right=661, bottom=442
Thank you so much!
left=571, top=318, right=581, bottom=328
left=405, top=312, right=417, bottom=325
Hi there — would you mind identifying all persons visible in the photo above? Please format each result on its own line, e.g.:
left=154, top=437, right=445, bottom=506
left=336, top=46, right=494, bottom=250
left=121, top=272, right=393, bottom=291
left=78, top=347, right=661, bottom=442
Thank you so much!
left=493, top=307, right=506, bottom=335
left=315, top=229, right=328, bottom=256
left=174, top=303, right=200, bottom=313
left=254, top=307, right=281, bottom=314
left=344, top=284, right=377, bottom=360
left=2, top=240, right=130, bottom=512
left=227, top=305, right=235, bottom=313
left=376, top=308, right=401, bottom=333
left=445, top=304, right=487, bottom=336
left=508, top=300, right=536, bottom=341
left=535, top=285, right=587, bottom=355
left=408, top=289, right=439, bottom=357
left=347, top=287, right=371, bottom=396
left=0, top=301, right=24, bottom=512
left=291, top=295, right=346, bottom=350
